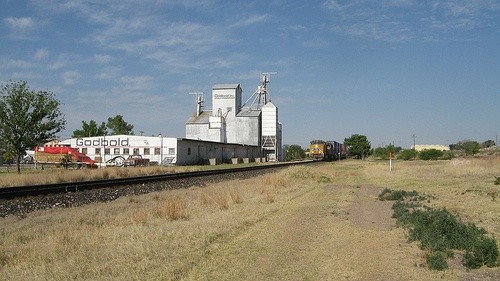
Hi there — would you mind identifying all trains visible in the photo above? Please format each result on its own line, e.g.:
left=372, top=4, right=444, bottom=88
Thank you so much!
left=309, top=139, right=347, bottom=162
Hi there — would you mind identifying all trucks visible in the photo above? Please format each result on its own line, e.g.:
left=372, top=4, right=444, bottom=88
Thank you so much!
left=35, top=146, right=99, bottom=170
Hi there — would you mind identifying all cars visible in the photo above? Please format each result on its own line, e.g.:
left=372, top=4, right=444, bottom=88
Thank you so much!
left=20, top=153, right=35, bottom=164
left=0, top=150, right=14, bottom=166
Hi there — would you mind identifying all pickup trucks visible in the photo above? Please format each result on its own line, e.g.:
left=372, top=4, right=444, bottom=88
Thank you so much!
left=122, top=154, right=150, bottom=167
